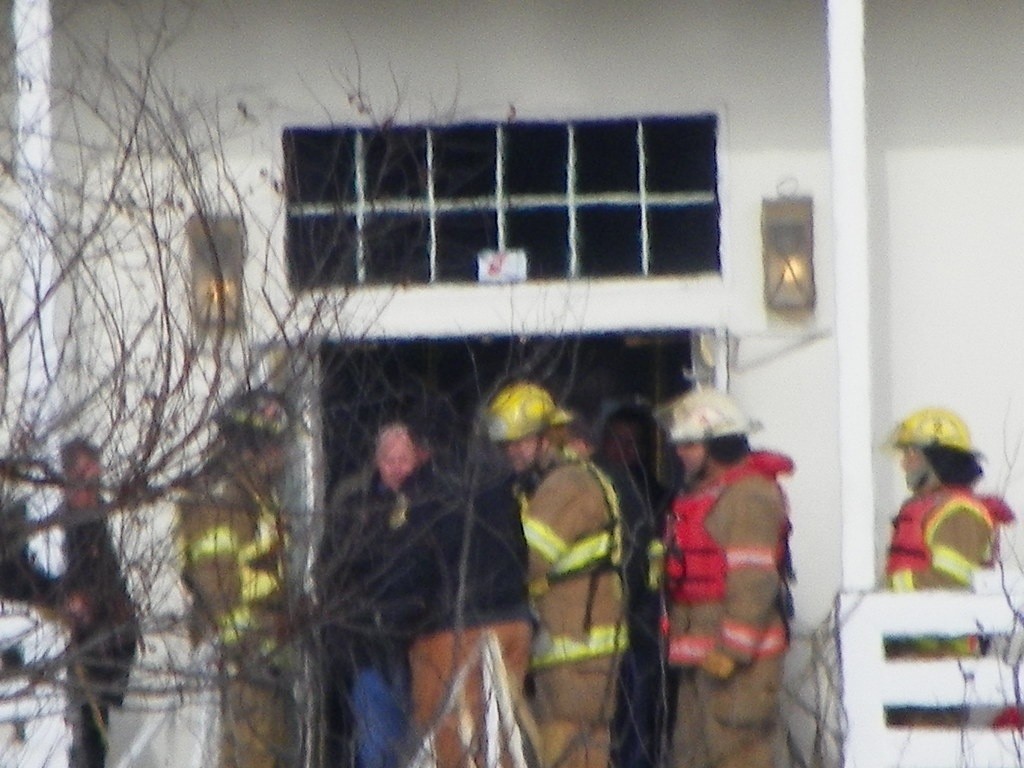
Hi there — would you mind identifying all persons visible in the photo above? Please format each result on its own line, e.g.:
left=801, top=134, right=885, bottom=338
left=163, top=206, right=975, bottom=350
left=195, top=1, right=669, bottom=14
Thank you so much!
left=882, top=411, right=1014, bottom=729
left=599, top=402, right=674, bottom=768
left=0, top=436, right=141, bottom=768
left=326, top=396, right=537, bottom=768
left=176, top=388, right=311, bottom=768
left=643, top=387, right=804, bottom=768
left=480, top=379, right=632, bottom=768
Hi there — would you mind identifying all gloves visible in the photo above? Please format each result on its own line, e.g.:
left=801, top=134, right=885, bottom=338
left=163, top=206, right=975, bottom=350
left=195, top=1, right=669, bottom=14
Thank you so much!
left=702, top=649, right=734, bottom=680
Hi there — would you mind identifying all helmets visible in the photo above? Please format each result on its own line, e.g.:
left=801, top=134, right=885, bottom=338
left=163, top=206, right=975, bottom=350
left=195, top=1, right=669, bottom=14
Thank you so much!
left=654, top=391, right=748, bottom=444
left=214, top=390, right=295, bottom=441
left=481, top=381, right=574, bottom=443
left=896, top=407, right=971, bottom=450
